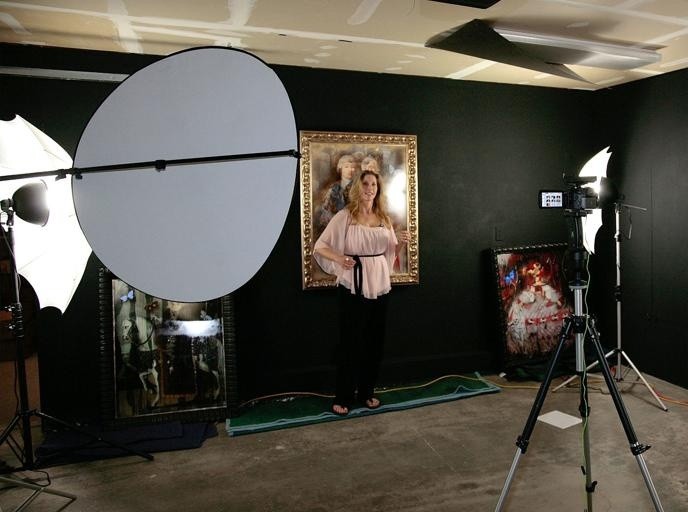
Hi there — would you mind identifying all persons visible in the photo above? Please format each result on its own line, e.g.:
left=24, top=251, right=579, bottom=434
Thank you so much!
left=318, top=150, right=382, bottom=236
left=312, top=170, right=411, bottom=417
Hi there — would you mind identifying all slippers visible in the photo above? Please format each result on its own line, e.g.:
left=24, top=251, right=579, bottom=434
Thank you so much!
left=332, top=396, right=381, bottom=415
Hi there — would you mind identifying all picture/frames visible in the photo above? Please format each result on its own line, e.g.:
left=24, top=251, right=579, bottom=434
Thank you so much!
left=492, top=242, right=575, bottom=369
left=298, top=129, right=419, bottom=292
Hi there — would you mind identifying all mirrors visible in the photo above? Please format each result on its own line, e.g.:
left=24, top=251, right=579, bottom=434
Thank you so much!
left=99, top=267, right=240, bottom=430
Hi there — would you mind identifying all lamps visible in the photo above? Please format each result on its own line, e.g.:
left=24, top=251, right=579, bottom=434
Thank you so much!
left=0, top=179, right=155, bottom=468
left=552, top=176, right=668, bottom=412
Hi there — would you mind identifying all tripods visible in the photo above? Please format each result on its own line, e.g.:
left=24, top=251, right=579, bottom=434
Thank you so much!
left=553, top=208, right=669, bottom=412
left=0, top=227, right=155, bottom=463
left=494, top=215, right=665, bottom=512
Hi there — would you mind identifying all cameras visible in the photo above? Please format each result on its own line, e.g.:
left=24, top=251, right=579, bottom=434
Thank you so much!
left=537, top=168, right=600, bottom=215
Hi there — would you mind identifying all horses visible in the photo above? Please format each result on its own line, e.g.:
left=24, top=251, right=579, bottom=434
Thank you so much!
left=122, top=316, right=224, bottom=407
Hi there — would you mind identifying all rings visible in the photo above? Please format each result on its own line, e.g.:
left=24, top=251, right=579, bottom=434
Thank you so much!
left=345, top=257, right=349, bottom=261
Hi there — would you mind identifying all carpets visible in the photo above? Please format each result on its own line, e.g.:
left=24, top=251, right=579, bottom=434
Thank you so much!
left=225, top=371, right=500, bottom=436
left=36, top=417, right=218, bottom=468
